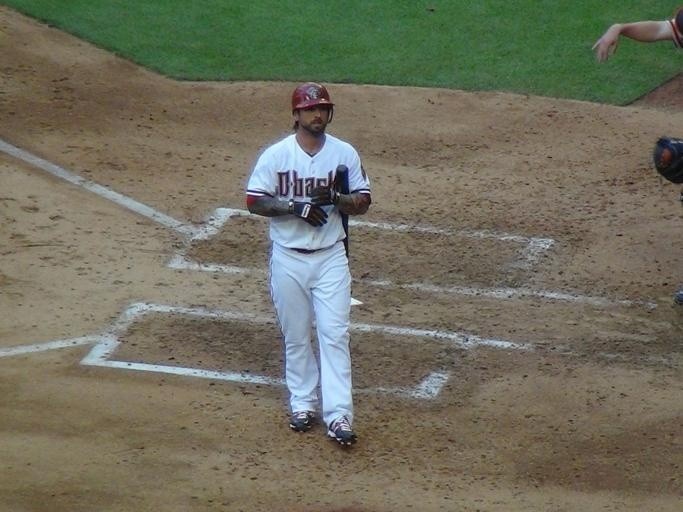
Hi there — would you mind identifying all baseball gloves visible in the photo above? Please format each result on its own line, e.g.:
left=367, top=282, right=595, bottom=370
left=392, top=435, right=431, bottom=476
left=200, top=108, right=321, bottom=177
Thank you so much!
left=655, top=136, right=683, bottom=184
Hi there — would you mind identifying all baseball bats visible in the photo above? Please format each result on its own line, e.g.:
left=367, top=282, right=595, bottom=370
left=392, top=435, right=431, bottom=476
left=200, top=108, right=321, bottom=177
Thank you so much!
left=336, top=165, right=348, bottom=259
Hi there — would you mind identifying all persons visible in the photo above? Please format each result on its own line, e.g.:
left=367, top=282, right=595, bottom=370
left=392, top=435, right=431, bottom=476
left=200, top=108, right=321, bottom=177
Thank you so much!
left=587, top=4, right=682, bottom=66
left=244, top=81, right=373, bottom=448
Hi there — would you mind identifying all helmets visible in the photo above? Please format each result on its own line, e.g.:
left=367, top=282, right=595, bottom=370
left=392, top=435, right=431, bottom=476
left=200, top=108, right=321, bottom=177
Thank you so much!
left=291, top=82, right=335, bottom=112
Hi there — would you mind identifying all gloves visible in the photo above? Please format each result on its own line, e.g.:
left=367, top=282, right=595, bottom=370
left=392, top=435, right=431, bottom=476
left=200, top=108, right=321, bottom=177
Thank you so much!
left=288, top=199, right=329, bottom=227
left=308, top=184, right=340, bottom=206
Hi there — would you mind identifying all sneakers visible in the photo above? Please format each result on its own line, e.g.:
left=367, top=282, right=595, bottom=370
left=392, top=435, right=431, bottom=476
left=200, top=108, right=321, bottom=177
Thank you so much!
left=327, top=415, right=359, bottom=446
left=288, top=411, right=316, bottom=433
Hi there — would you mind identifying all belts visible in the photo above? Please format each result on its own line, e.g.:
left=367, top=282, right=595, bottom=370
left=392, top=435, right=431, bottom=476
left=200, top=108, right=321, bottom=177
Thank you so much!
left=291, top=247, right=322, bottom=253
